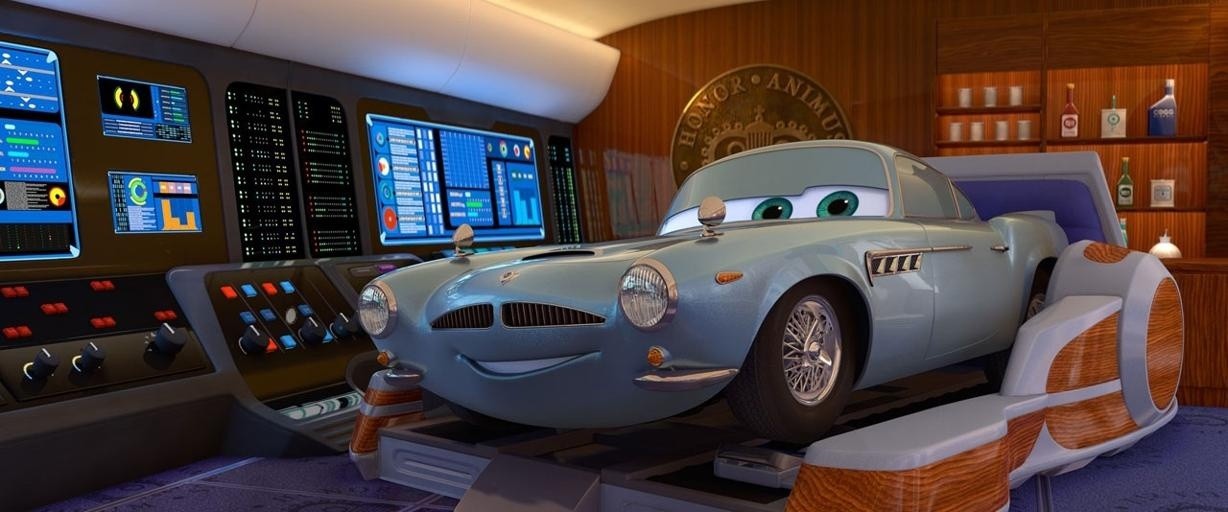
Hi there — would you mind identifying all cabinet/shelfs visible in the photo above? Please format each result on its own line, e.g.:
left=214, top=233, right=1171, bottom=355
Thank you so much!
left=933, top=14, right=1222, bottom=256
left=1157, top=255, right=1228, bottom=411
